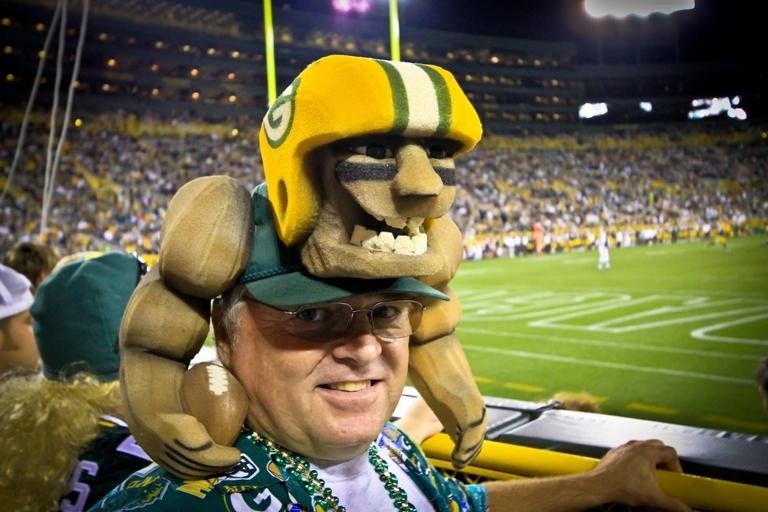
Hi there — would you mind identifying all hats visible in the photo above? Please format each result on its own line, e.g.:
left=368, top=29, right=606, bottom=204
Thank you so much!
left=30, top=253, right=147, bottom=382
left=241, top=182, right=452, bottom=306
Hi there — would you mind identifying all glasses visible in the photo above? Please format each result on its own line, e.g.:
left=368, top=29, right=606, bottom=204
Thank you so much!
left=241, top=296, right=423, bottom=346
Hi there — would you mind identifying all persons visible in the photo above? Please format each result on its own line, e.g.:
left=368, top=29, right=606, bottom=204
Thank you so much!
left=79, top=178, right=689, bottom=510
left=0, top=261, right=41, bottom=381
left=0, top=247, right=151, bottom=512
